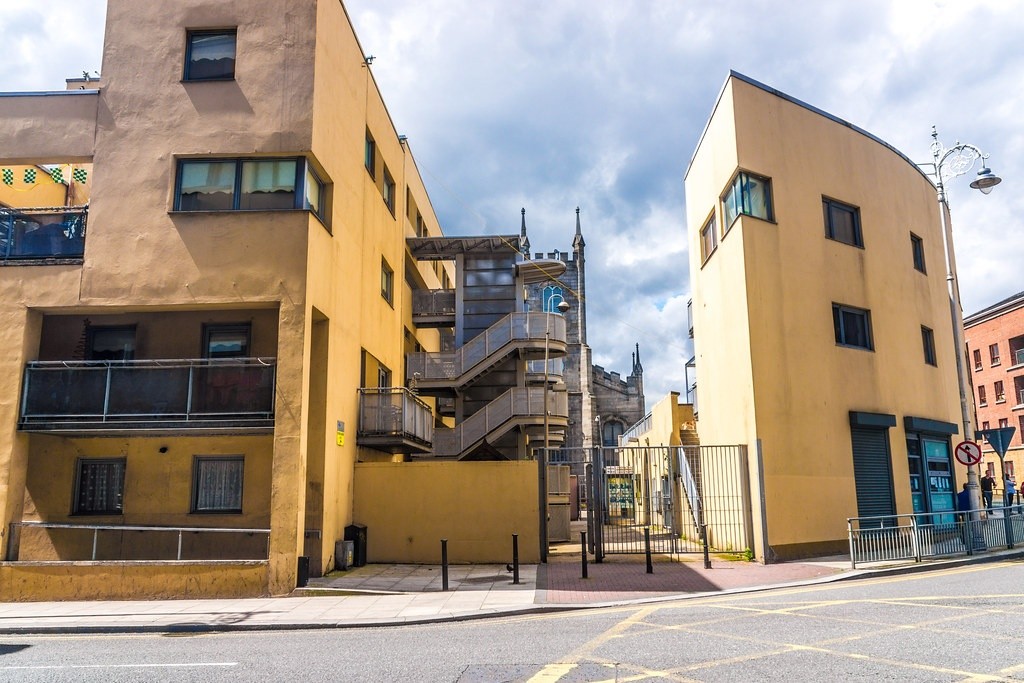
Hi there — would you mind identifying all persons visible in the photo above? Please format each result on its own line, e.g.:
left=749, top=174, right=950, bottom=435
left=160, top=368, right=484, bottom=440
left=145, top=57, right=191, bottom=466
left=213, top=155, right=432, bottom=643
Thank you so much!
left=1006, top=474, right=1017, bottom=515
left=957, top=483, right=971, bottom=545
left=981, top=470, right=997, bottom=515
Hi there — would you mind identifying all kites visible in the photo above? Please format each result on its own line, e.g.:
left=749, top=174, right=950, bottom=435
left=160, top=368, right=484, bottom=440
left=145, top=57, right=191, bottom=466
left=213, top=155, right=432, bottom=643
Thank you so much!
left=2, top=165, right=89, bottom=201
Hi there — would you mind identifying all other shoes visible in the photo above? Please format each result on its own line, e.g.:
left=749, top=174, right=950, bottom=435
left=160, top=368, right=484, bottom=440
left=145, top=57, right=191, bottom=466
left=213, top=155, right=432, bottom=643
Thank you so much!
left=1009, top=512, right=1016, bottom=515
left=989, top=511, right=993, bottom=514
